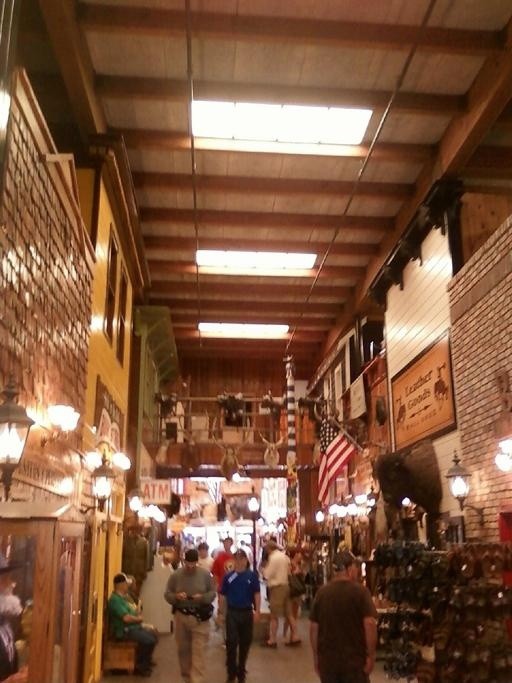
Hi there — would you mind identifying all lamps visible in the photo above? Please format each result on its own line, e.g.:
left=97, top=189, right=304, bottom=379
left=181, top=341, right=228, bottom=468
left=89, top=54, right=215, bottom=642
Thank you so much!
left=93, top=433, right=134, bottom=472
left=40, top=397, right=80, bottom=448
left=446, top=446, right=484, bottom=524
left=365, top=485, right=381, bottom=506
left=81, top=432, right=131, bottom=514
left=0, top=363, right=38, bottom=499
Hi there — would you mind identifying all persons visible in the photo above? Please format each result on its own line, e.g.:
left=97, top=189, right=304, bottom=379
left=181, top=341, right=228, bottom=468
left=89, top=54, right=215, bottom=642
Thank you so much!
left=126, top=575, right=160, bottom=665
left=164, top=537, right=307, bottom=683
left=108, top=573, right=156, bottom=677
left=308, top=551, right=378, bottom=683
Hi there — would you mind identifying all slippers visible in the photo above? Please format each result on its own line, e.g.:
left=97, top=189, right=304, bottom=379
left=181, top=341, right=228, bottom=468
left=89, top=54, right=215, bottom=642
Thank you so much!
left=284, top=639, right=301, bottom=646
left=259, top=640, right=278, bottom=648
left=373, top=541, right=512, bottom=683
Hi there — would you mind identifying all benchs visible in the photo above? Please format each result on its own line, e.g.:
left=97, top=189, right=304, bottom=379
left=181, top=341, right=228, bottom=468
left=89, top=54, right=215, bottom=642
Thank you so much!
left=103, top=638, right=139, bottom=674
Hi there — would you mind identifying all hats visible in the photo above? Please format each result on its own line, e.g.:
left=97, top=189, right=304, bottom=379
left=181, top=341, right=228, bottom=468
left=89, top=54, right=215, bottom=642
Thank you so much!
left=113, top=573, right=133, bottom=585
left=332, top=549, right=363, bottom=571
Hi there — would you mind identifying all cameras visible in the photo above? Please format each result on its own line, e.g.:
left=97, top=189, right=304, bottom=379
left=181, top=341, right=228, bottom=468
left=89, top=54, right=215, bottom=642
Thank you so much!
left=187, top=596, right=194, bottom=600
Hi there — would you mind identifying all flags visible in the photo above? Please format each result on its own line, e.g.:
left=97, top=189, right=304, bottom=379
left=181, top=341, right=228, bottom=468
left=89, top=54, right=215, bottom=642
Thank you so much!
left=317, top=410, right=357, bottom=508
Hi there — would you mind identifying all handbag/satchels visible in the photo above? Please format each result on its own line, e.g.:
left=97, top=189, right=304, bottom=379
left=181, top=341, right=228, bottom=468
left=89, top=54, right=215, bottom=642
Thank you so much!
left=288, top=574, right=308, bottom=597
left=194, top=603, right=215, bottom=622
